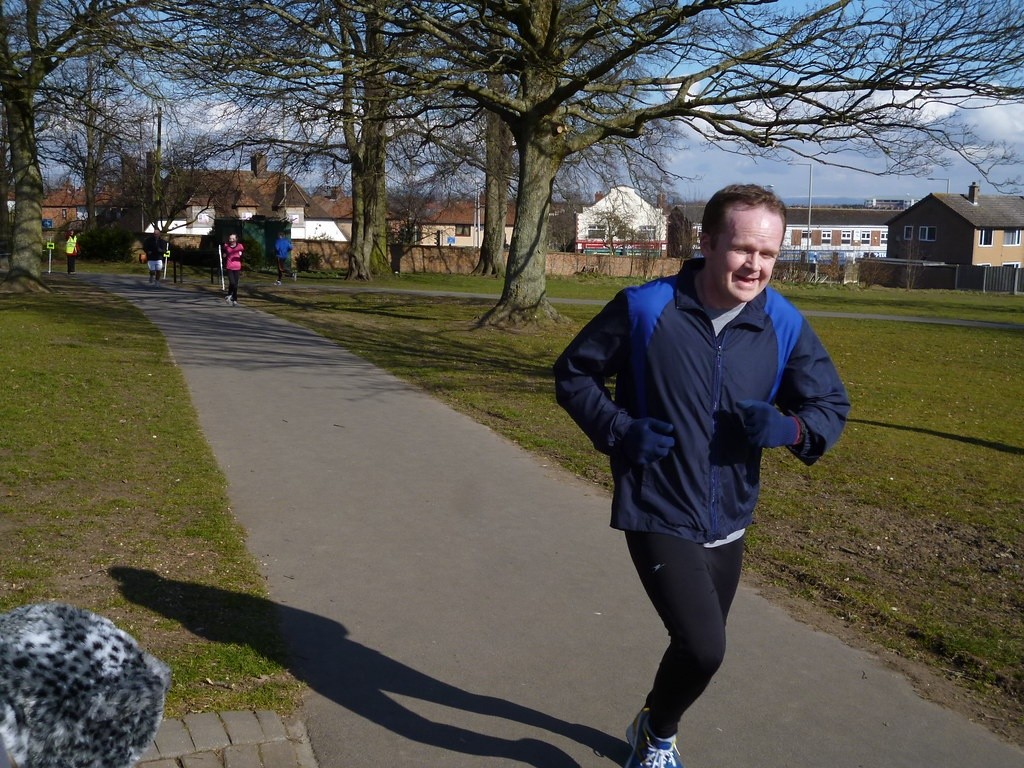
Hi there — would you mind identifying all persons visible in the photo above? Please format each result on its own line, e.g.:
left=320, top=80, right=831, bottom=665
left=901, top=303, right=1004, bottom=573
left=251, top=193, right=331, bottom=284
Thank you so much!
left=273, top=231, right=296, bottom=285
left=66, top=228, right=81, bottom=274
left=552, top=183, right=851, bottom=768
left=143, top=229, right=167, bottom=289
left=218, top=233, right=244, bottom=305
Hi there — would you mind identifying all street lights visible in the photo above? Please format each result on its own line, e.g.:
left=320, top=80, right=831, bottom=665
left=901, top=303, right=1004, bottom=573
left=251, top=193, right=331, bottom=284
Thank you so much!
left=926, top=177, right=950, bottom=194
left=779, top=162, right=813, bottom=250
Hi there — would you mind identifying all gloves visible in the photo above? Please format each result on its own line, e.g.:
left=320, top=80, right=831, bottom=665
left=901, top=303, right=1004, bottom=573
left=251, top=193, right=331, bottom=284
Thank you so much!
left=734, top=398, right=801, bottom=450
left=620, top=417, right=675, bottom=471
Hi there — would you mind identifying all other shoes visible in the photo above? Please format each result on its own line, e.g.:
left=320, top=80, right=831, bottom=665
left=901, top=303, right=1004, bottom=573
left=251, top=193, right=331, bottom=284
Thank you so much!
left=224, top=295, right=232, bottom=304
left=68, top=272, right=76, bottom=277
left=149, top=281, right=160, bottom=289
left=232, top=301, right=240, bottom=308
left=273, top=280, right=281, bottom=286
left=292, top=272, right=297, bottom=282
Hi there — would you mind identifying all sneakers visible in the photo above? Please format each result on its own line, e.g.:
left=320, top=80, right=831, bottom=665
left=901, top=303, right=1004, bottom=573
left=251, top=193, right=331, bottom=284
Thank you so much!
left=625, top=705, right=684, bottom=768
